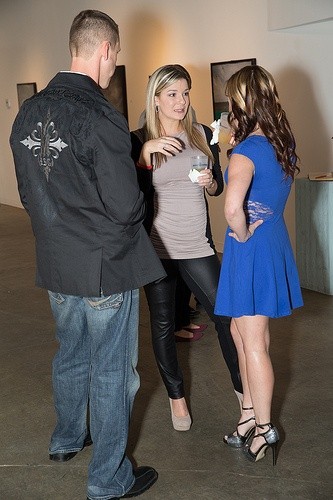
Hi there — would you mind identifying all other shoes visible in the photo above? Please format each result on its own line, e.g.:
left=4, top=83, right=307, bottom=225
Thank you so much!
left=49, top=425, right=92, bottom=462
left=175, top=324, right=208, bottom=342
left=87, top=465, right=158, bottom=500
left=188, top=311, right=200, bottom=319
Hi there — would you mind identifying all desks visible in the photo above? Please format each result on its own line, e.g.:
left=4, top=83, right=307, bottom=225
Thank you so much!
left=294, top=177, right=333, bottom=296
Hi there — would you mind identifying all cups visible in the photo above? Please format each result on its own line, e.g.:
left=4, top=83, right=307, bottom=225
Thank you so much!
left=217, top=111, right=232, bottom=145
left=191, top=155, right=208, bottom=171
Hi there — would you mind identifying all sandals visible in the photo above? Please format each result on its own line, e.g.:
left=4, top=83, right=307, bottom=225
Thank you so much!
left=223, top=416, right=280, bottom=466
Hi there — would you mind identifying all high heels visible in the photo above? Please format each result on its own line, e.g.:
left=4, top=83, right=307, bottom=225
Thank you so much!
left=168, top=397, right=191, bottom=431
left=234, top=390, right=243, bottom=414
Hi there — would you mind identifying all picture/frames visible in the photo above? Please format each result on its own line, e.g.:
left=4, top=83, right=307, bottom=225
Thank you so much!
left=17, top=82, right=37, bottom=110
left=210, top=58, right=257, bottom=121
left=102, top=65, right=128, bottom=123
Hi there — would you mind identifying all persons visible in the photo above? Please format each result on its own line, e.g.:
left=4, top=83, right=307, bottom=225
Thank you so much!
left=10, top=9, right=168, bottom=500
left=127, top=64, right=244, bottom=432
left=221, top=66, right=300, bottom=466
